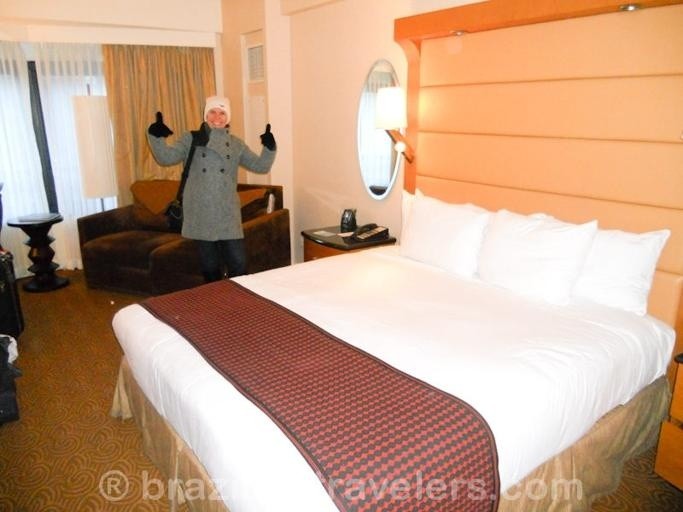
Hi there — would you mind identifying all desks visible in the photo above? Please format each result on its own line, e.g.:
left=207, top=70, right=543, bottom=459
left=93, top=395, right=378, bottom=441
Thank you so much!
left=6, top=214, right=70, bottom=293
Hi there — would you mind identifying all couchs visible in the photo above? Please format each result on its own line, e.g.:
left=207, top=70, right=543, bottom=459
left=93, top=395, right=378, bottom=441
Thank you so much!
left=77, top=177, right=290, bottom=296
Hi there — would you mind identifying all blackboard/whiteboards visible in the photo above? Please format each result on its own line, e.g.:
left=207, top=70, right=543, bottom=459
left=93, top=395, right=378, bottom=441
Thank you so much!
left=342, top=222, right=389, bottom=244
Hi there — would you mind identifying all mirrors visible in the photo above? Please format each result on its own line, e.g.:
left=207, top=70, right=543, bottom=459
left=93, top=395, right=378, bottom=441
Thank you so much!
left=355, top=58, right=404, bottom=200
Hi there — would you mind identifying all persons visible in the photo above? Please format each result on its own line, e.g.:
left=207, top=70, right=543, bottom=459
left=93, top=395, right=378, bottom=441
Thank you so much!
left=141, top=92, right=278, bottom=287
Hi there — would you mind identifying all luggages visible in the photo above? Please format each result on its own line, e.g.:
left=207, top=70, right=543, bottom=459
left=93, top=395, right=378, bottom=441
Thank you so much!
left=0, top=251, right=25, bottom=336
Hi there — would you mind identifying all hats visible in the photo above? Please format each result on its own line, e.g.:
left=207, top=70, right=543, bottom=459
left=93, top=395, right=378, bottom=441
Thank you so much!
left=204, top=96, right=231, bottom=126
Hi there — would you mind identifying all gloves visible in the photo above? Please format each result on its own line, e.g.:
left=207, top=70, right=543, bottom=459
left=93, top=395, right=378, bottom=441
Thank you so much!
left=149, top=112, right=173, bottom=138
left=260, top=124, right=276, bottom=151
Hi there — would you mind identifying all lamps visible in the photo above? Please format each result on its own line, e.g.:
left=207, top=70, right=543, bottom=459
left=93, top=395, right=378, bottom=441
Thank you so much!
left=371, top=84, right=414, bottom=166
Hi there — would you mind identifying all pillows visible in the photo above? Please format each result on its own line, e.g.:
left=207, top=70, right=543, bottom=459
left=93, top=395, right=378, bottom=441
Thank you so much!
left=398, top=187, right=495, bottom=285
left=400, top=189, right=490, bottom=279
left=475, top=208, right=598, bottom=305
left=573, top=227, right=670, bottom=318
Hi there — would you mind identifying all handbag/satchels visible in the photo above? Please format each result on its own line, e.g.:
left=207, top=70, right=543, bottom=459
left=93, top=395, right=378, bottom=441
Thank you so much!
left=165, top=201, right=183, bottom=232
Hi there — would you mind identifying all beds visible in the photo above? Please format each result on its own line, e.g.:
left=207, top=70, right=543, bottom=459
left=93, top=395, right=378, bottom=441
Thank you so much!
left=106, top=192, right=676, bottom=511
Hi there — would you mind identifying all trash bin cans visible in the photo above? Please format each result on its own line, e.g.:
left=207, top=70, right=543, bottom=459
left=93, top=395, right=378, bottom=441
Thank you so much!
left=0, top=333, right=22, bottom=424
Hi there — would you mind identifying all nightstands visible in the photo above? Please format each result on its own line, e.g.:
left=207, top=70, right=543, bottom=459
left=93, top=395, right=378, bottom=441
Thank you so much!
left=301, top=223, right=396, bottom=263
left=654, top=354, right=683, bottom=492
